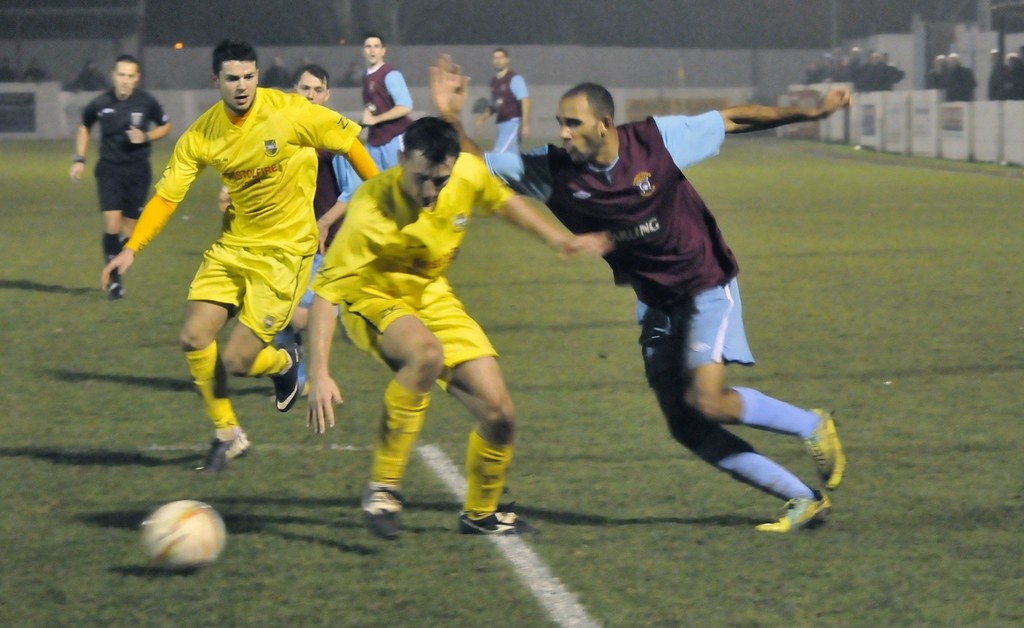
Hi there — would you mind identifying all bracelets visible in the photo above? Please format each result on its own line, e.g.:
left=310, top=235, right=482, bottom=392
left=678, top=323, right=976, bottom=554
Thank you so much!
left=143, top=131, right=150, bottom=143
left=72, top=154, right=86, bottom=164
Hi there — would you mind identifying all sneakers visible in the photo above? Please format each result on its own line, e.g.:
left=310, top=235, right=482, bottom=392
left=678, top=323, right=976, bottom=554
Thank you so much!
left=458, top=504, right=538, bottom=537
left=754, top=491, right=834, bottom=533
left=197, top=430, right=249, bottom=473
left=268, top=342, right=303, bottom=410
left=109, top=282, right=123, bottom=299
left=361, top=482, right=407, bottom=539
left=803, top=407, right=846, bottom=490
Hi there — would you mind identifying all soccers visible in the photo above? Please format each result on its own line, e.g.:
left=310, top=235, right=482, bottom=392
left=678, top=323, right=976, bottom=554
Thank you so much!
left=145, top=498, right=228, bottom=570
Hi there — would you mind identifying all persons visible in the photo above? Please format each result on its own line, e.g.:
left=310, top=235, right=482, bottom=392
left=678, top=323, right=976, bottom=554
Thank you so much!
left=101, top=35, right=381, bottom=480
left=68, top=52, right=171, bottom=300
left=802, top=47, right=1024, bottom=101
left=355, top=32, right=415, bottom=178
left=431, top=54, right=856, bottom=536
left=473, top=45, right=531, bottom=154
left=303, top=116, right=611, bottom=541
